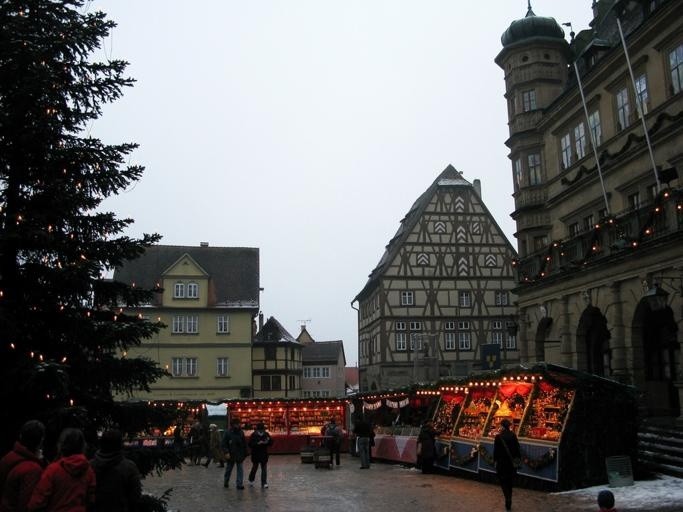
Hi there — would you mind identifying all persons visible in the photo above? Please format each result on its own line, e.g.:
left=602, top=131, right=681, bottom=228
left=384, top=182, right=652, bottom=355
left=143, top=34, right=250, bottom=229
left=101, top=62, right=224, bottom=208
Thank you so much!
left=596, top=489, right=616, bottom=511
left=25, top=427, right=97, bottom=512
left=351, top=417, right=372, bottom=470
left=247, top=421, right=272, bottom=490
left=323, top=418, right=342, bottom=468
left=417, top=419, right=442, bottom=474
left=220, top=418, right=249, bottom=491
left=491, top=419, right=521, bottom=512
left=201, top=424, right=225, bottom=469
left=88, top=429, right=142, bottom=511
left=171, top=422, right=187, bottom=465
left=187, top=420, right=204, bottom=465
left=0, top=419, right=47, bottom=510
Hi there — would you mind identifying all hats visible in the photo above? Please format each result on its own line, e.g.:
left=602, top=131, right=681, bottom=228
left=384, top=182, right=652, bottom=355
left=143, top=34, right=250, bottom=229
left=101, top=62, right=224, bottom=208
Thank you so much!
left=252, top=423, right=268, bottom=431
left=500, top=420, right=510, bottom=428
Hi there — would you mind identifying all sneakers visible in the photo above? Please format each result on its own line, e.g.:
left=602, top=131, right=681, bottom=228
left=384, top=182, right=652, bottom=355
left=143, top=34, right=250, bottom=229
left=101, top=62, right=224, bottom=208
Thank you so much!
left=236, top=485, right=244, bottom=490
left=223, top=482, right=229, bottom=488
left=248, top=481, right=255, bottom=488
left=261, top=483, right=269, bottom=489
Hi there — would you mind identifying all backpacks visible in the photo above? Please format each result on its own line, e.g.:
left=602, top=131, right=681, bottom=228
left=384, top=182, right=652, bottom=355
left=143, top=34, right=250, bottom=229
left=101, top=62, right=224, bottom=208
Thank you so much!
left=326, top=424, right=338, bottom=441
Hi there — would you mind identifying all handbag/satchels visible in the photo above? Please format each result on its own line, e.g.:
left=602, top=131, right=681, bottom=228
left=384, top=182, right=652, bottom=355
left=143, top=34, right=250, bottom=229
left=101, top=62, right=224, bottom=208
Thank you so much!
left=512, top=457, right=521, bottom=470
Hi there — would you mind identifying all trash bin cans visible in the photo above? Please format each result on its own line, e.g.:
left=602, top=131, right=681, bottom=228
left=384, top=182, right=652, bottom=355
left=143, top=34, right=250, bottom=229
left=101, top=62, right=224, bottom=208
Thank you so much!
left=606, top=457, right=634, bottom=488
